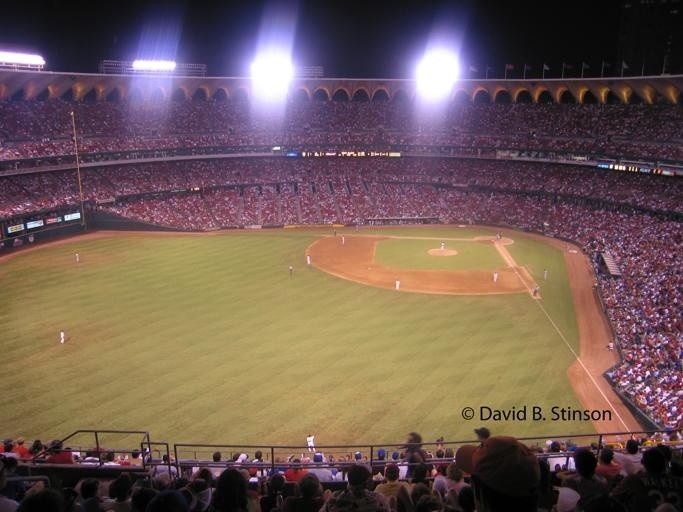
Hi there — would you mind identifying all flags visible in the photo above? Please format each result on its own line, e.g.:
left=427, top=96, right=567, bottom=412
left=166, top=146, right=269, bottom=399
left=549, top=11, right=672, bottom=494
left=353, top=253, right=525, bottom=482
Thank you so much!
left=564, top=63, right=569, bottom=68
left=544, top=64, right=550, bottom=70
left=506, top=64, right=513, bottom=69
left=602, top=62, right=609, bottom=68
left=469, top=64, right=478, bottom=73
left=622, top=61, right=629, bottom=68
left=525, top=65, right=531, bottom=70
left=487, top=64, right=495, bottom=70
left=583, top=63, right=589, bottom=68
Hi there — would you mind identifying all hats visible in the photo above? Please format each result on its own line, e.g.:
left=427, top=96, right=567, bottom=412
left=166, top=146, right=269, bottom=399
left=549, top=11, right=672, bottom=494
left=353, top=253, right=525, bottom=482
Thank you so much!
left=330, top=469, right=338, bottom=475
left=314, top=449, right=399, bottom=462
left=148, top=490, right=187, bottom=512
left=455, top=437, right=542, bottom=495
left=0, top=454, right=17, bottom=479
left=17, top=437, right=24, bottom=443
left=474, top=428, right=490, bottom=440
